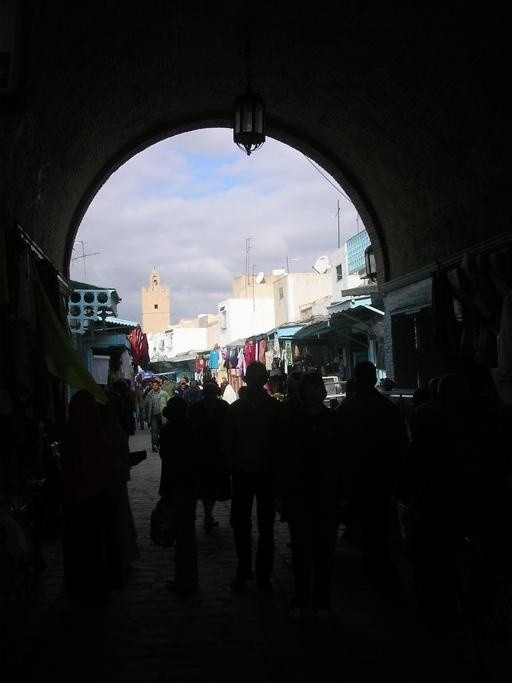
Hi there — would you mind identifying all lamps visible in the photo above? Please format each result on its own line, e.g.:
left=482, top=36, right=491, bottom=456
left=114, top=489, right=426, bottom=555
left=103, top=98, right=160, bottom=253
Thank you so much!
left=232, top=0, right=266, bottom=157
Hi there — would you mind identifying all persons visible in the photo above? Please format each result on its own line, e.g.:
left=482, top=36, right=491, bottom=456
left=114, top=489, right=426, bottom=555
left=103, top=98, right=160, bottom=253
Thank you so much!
left=1, top=351, right=512, bottom=618
